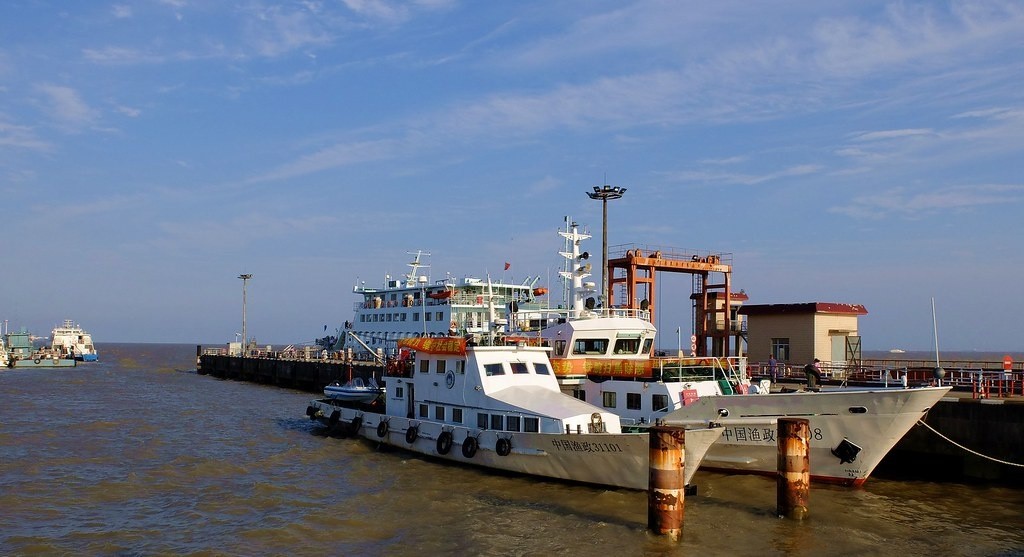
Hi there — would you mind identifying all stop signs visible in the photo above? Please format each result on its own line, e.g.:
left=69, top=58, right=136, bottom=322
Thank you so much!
left=1001, top=355, right=1014, bottom=369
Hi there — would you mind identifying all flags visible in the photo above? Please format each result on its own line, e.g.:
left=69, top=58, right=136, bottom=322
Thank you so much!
left=504, top=262, right=510, bottom=270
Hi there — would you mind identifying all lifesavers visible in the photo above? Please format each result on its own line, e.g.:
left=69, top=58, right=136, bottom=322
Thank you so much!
left=394, top=301, right=398, bottom=306
left=305, top=406, right=313, bottom=416
left=350, top=417, right=362, bottom=434
left=328, top=410, right=340, bottom=428
left=367, top=302, right=371, bottom=308
left=309, top=407, right=319, bottom=421
left=377, top=420, right=388, bottom=438
left=436, top=431, right=453, bottom=456
left=402, top=300, right=406, bottom=306
left=381, top=302, right=385, bottom=307
left=410, top=300, right=414, bottom=306
left=388, top=301, right=392, bottom=307
left=418, top=300, right=422, bottom=306
left=405, top=426, right=418, bottom=444
left=462, top=437, right=478, bottom=458
left=495, top=438, right=511, bottom=456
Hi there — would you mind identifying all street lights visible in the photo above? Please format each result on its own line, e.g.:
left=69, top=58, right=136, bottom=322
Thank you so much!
left=237, top=273, right=254, bottom=357
left=585, top=171, right=627, bottom=316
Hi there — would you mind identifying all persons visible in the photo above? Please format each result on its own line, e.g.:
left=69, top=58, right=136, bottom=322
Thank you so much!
left=766, top=354, right=779, bottom=388
left=802, top=358, right=824, bottom=388
left=654, top=350, right=665, bottom=356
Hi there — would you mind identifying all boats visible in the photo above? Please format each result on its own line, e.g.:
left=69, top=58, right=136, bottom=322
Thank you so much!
left=0, top=319, right=76, bottom=367
left=310, top=220, right=954, bottom=487
left=44, top=319, right=99, bottom=362
left=305, top=213, right=729, bottom=492
left=324, top=377, right=382, bottom=404
left=429, top=290, right=458, bottom=299
